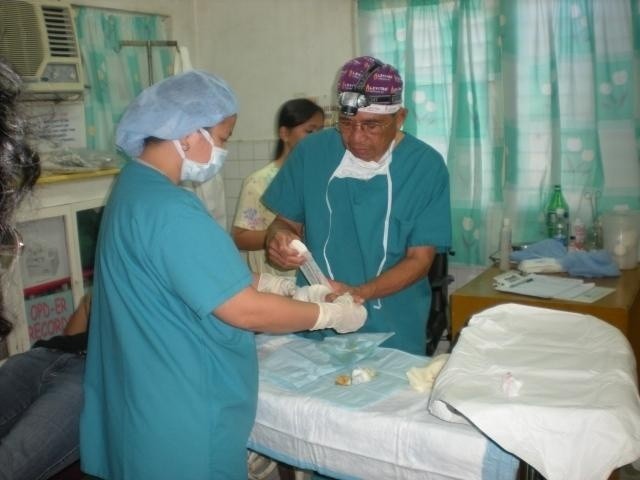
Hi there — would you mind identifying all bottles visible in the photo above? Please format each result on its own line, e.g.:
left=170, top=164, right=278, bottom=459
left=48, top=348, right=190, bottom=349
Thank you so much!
left=546, top=185, right=570, bottom=246
left=500, top=219, right=511, bottom=272
left=552, top=210, right=566, bottom=246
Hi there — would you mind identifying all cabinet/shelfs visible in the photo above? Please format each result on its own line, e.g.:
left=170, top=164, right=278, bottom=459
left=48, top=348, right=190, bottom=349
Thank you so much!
left=2, top=165, right=195, bottom=354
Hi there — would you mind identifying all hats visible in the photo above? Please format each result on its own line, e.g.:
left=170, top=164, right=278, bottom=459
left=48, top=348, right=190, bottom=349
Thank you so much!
left=328, top=56, right=404, bottom=115
left=113, top=69, right=239, bottom=160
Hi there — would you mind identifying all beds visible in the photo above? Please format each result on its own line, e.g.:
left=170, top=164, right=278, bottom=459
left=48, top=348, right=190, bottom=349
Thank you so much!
left=246, top=335, right=523, bottom=479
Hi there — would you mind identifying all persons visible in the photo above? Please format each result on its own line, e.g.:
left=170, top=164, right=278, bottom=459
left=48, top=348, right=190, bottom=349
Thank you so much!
left=0, top=293, right=92, bottom=480
left=79, top=70, right=368, bottom=480
left=232, top=99, right=324, bottom=279
left=259, top=56, right=451, bottom=358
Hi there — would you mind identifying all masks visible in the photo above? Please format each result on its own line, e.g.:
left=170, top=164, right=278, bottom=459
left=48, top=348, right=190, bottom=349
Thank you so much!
left=331, top=139, right=396, bottom=181
left=172, top=127, right=228, bottom=184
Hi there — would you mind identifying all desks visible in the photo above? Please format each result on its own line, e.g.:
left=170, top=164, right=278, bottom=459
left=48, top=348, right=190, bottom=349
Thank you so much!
left=450, top=264, right=638, bottom=350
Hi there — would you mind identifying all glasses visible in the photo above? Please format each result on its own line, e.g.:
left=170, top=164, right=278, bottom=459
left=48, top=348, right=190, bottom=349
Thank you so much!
left=334, top=116, right=398, bottom=135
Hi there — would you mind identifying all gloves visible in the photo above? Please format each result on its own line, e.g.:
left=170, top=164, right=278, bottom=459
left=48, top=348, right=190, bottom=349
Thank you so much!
left=257, top=271, right=331, bottom=304
left=309, top=292, right=367, bottom=334
